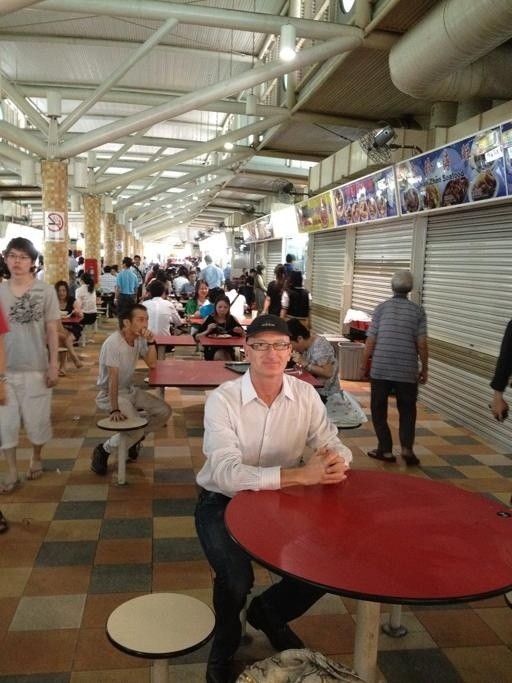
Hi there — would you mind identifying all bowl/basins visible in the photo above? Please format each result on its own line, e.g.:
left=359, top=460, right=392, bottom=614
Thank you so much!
left=60, top=311, right=68, bottom=315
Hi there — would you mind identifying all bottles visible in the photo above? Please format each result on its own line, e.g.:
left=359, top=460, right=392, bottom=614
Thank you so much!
left=257, top=296, right=263, bottom=313
left=319, top=197, right=329, bottom=228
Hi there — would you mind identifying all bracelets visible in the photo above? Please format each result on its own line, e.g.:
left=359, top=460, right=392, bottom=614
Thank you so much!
left=109, top=410, right=121, bottom=414
left=147, top=340, right=156, bottom=346
left=0, top=374, right=10, bottom=384
left=304, top=362, right=312, bottom=371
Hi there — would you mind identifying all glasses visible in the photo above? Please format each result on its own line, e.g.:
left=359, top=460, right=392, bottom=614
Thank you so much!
left=245, top=340, right=292, bottom=351
left=6, top=251, right=31, bottom=260
left=198, top=287, right=208, bottom=291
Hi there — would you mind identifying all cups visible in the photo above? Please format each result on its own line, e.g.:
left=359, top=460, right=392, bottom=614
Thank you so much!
left=252, top=310, right=258, bottom=320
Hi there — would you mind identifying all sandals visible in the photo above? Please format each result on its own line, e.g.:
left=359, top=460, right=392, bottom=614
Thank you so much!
left=58, top=365, right=66, bottom=376
left=0, top=510, right=7, bottom=534
left=73, top=359, right=84, bottom=368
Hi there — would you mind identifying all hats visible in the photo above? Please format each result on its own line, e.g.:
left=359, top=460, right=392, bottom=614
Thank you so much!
left=286, top=254, right=296, bottom=262
left=248, top=314, right=294, bottom=336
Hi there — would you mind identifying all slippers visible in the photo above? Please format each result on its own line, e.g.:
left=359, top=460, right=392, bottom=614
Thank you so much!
left=367, top=448, right=397, bottom=462
left=0, top=475, right=20, bottom=496
left=25, top=459, right=43, bottom=479
left=401, top=450, right=421, bottom=466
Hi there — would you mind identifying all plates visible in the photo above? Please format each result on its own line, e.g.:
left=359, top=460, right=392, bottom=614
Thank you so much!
left=470, top=130, right=499, bottom=173
left=208, top=333, right=232, bottom=339
left=367, top=198, right=377, bottom=218
left=420, top=182, right=440, bottom=210
left=333, top=189, right=344, bottom=217
left=467, top=173, right=499, bottom=202
left=376, top=196, right=386, bottom=217
left=360, top=201, right=368, bottom=220
left=402, top=186, right=420, bottom=212
left=351, top=204, right=360, bottom=221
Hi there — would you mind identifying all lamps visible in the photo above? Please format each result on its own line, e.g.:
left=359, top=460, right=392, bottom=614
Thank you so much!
left=245, top=0, right=257, bottom=117
left=223, top=96, right=234, bottom=150
left=279, top=24, right=295, bottom=62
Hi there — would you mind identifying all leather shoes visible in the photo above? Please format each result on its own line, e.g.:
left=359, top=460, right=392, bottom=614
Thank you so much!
left=246, top=595, right=304, bottom=652
left=206, top=625, right=241, bottom=683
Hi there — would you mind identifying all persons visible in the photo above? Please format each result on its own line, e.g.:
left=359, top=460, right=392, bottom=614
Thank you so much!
left=245, top=269, right=257, bottom=314
left=130, top=255, right=146, bottom=302
left=286, top=317, right=340, bottom=404
left=90, top=304, right=172, bottom=474
left=55, top=281, right=84, bottom=377
left=262, top=264, right=287, bottom=317
left=239, top=268, right=248, bottom=280
left=141, top=281, right=183, bottom=337
left=185, top=280, right=211, bottom=335
left=68, top=249, right=84, bottom=298
left=178, top=257, right=200, bottom=278
left=199, top=254, right=225, bottom=289
left=0, top=249, right=11, bottom=535
left=284, top=255, right=296, bottom=275
left=280, top=271, right=312, bottom=330
left=114, top=257, right=140, bottom=330
left=225, top=282, right=247, bottom=332
left=222, top=262, right=231, bottom=291
left=142, top=263, right=162, bottom=300
left=192, top=294, right=243, bottom=360
left=0, top=237, right=60, bottom=496
left=181, top=271, right=198, bottom=300
left=72, top=272, right=98, bottom=324
left=0, top=250, right=45, bottom=282
left=99, top=265, right=116, bottom=318
left=195, top=287, right=225, bottom=321
left=253, top=265, right=268, bottom=317
left=111, top=265, right=119, bottom=277
left=163, top=259, right=177, bottom=299
left=360, top=269, right=429, bottom=466
left=194, top=311, right=352, bottom=682
left=173, top=269, right=189, bottom=301
left=490, top=320, right=511, bottom=605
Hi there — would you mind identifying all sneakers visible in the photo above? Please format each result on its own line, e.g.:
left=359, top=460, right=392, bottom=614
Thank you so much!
left=128, top=435, right=145, bottom=458
left=90, top=442, right=110, bottom=474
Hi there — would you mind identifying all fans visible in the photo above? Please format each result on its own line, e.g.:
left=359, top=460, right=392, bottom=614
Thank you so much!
left=355, top=126, right=423, bottom=166
left=271, top=177, right=312, bottom=205
left=239, top=202, right=268, bottom=222
left=194, top=221, right=238, bottom=242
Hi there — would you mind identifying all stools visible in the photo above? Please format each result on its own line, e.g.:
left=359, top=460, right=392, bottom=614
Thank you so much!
left=131, top=368, right=166, bottom=412
left=54, top=296, right=111, bottom=373
left=95, top=415, right=149, bottom=488
left=335, top=424, right=365, bottom=430
left=105, top=592, right=215, bottom=683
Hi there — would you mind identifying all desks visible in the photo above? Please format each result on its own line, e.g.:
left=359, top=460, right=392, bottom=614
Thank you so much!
left=199, top=334, right=249, bottom=359
left=225, top=470, right=511, bottom=683
left=180, top=316, right=258, bottom=330
left=153, top=335, right=195, bottom=361
left=148, top=360, right=324, bottom=427
